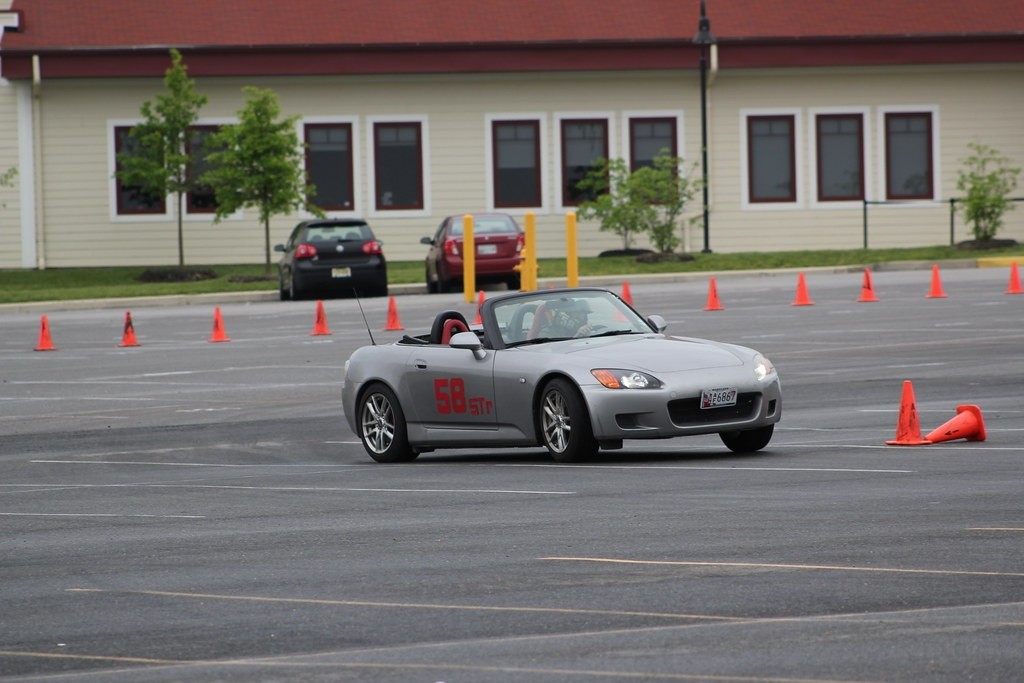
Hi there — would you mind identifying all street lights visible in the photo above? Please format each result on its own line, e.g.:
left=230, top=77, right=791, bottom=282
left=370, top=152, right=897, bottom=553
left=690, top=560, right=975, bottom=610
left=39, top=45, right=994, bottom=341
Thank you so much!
left=693, top=0, right=718, bottom=256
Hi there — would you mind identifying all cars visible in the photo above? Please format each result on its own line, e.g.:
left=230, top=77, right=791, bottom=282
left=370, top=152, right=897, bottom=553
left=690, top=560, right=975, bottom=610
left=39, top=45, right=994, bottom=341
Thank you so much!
left=273, top=217, right=388, bottom=301
left=420, top=213, right=526, bottom=294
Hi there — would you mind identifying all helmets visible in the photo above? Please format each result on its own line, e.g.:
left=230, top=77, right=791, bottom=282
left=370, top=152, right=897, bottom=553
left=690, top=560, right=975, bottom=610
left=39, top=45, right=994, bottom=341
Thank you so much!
left=544, top=299, right=593, bottom=332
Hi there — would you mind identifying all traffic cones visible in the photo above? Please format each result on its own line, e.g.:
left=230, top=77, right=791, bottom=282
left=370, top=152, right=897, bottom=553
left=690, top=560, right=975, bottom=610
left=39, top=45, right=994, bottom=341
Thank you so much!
left=309, top=299, right=334, bottom=336
left=923, top=403, right=986, bottom=444
left=856, top=267, right=881, bottom=302
left=381, top=293, right=406, bottom=330
left=614, top=282, right=637, bottom=320
left=788, top=271, right=818, bottom=307
left=471, top=289, right=486, bottom=325
left=1003, top=260, right=1024, bottom=295
left=31, top=314, right=58, bottom=351
left=208, top=304, right=231, bottom=342
left=702, top=275, right=725, bottom=311
left=925, top=263, right=949, bottom=299
left=884, top=379, right=933, bottom=446
left=117, top=310, right=143, bottom=347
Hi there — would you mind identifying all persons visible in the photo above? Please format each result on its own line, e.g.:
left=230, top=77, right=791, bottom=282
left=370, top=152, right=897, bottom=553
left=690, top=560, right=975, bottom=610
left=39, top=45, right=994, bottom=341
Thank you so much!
left=538, top=298, right=594, bottom=338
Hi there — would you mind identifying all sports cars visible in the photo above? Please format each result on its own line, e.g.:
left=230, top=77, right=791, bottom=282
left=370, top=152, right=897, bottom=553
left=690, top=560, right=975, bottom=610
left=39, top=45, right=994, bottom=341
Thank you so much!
left=342, top=285, right=784, bottom=465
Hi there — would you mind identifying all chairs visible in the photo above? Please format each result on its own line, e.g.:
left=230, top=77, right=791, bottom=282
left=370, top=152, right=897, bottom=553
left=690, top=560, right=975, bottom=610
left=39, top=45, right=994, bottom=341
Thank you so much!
left=311, top=234, right=324, bottom=241
left=346, top=232, right=360, bottom=238
left=330, top=236, right=341, bottom=240
left=529, top=303, right=557, bottom=340
left=442, top=320, right=470, bottom=345
